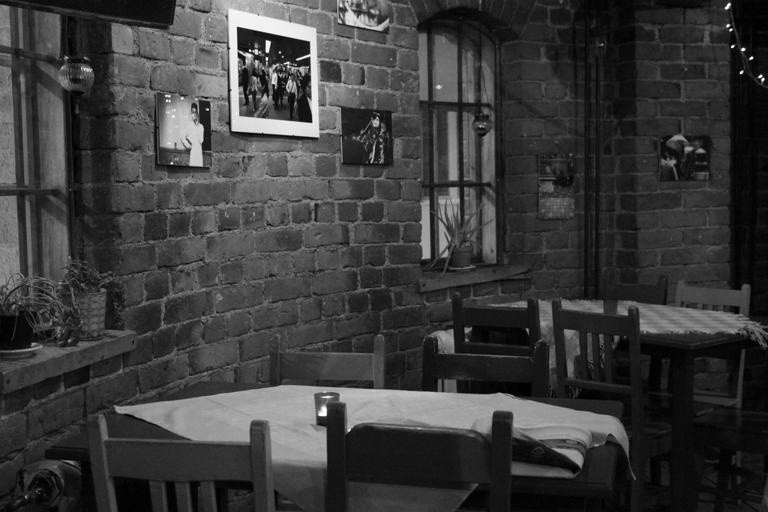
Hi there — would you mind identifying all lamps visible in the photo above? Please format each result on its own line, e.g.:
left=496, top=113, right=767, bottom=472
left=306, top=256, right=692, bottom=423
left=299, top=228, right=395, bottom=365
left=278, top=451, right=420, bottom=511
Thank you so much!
left=57, top=13, right=96, bottom=115
left=472, top=67, right=493, bottom=137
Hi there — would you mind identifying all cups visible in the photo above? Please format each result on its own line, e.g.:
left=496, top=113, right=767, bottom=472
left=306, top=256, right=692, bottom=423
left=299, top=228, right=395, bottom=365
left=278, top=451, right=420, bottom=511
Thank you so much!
left=313, top=391, right=341, bottom=426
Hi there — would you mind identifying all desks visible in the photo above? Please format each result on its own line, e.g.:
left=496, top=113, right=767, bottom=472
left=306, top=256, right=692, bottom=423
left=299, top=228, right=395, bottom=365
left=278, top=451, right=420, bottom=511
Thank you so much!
left=486, top=299, right=768, bottom=512
left=45, top=381, right=625, bottom=512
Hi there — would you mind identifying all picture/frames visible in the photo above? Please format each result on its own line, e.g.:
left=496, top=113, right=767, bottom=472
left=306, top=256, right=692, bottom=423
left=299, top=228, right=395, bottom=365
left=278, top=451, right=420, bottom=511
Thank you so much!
left=227, top=7, right=320, bottom=139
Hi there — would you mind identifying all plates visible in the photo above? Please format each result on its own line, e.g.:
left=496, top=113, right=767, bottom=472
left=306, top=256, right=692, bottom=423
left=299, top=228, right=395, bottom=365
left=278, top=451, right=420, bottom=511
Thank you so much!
left=0, top=342, right=44, bottom=362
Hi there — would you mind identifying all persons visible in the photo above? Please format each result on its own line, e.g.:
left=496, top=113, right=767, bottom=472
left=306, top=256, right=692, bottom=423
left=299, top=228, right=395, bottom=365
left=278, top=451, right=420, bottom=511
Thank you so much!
left=241, top=61, right=312, bottom=122
left=179, top=103, right=205, bottom=167
left=357, top=111, right=390, bottom=164
left=662, top=141, right=708, bottom=182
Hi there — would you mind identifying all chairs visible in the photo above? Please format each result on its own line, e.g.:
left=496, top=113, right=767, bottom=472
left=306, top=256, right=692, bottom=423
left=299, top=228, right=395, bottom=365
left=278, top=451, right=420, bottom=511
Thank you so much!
left=268, top=334, right=386, bottom=389
left=85, top=413, right=275, bottom=512
left=648, top=278, right=752, bottom=510
left=452, top=292, right=541, bottom=393
left=552, top=298, right=673, bottom=512
left=422, top=336, right=549, bottom=398
left=578, top=268, right=667, bottom=486
left=327, top=403, right=513, bottom=512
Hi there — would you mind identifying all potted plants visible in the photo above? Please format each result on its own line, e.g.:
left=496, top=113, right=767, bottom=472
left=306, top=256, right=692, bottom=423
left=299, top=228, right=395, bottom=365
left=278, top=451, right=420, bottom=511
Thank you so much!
left=426, top=196, right=493, bottom=277
left=58, top=256, right=118, bottom=341
left=0, top=271, right=68, bottom=350
